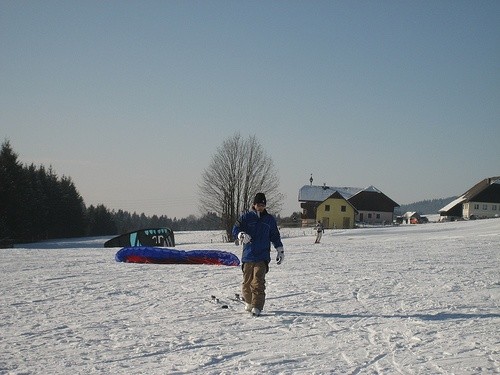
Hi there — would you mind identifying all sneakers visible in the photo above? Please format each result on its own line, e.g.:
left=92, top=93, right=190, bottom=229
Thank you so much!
left=245, top=303, right=252, bottom=311
left=252, top=308, right=261, bottom=316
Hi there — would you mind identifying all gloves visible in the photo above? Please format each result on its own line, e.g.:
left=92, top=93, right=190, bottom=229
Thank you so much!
left=238, top=232, right=252, bottom=244
left=275, top=247, right=285, bottom=265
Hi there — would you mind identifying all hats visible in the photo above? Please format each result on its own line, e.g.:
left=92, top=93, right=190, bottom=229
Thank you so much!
left=253, top=192, right=266, bottom=204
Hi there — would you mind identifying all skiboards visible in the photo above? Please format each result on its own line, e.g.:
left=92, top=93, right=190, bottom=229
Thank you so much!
left=208, top=292, right=250, bottom=311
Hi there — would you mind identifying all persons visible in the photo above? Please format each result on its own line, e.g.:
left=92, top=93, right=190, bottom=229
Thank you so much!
left=313, top=221, right=324, bottom=244
left=233, top=192, right=285, bottom=316
